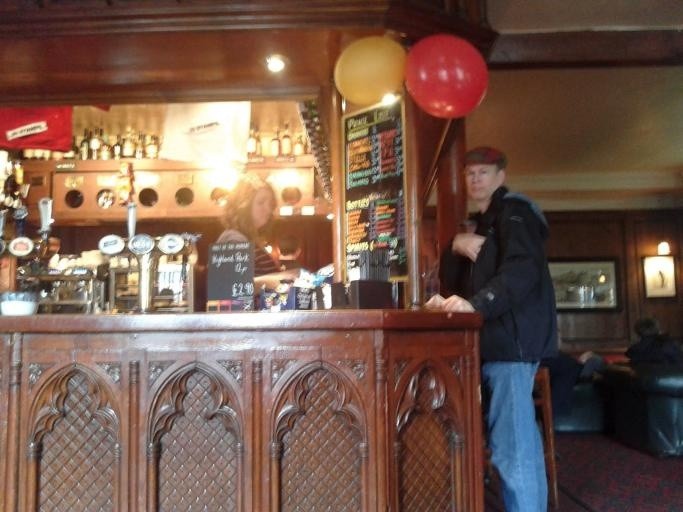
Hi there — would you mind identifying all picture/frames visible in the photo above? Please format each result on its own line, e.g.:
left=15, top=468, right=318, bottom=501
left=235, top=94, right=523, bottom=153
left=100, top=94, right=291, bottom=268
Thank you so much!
left=546, top=257, right=622, bottom=312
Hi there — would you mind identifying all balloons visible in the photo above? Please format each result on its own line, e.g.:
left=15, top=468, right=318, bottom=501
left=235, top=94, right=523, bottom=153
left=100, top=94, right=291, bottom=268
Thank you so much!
left=402, top=32, right=489, bottom=121
left=333, top=34, right=407, bottom=104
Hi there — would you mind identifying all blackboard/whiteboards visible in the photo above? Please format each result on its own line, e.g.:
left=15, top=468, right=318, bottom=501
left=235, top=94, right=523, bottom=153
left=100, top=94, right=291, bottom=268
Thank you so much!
left=341, top=99, right=408, bottom=285
left=207, top=241, right=254, bottom=311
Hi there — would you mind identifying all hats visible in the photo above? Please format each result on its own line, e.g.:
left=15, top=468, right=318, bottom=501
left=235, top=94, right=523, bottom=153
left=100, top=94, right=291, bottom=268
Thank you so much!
left=463, top=146, right=505, bottom=164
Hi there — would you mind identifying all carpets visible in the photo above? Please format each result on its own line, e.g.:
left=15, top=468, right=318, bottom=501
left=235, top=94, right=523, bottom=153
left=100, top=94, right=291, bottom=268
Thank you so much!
left=542, top=432, right=683, bottom=512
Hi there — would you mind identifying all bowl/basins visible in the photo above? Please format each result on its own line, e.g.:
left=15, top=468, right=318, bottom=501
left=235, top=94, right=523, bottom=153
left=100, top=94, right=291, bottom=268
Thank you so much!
left=1, top=300, right=39, bottom=318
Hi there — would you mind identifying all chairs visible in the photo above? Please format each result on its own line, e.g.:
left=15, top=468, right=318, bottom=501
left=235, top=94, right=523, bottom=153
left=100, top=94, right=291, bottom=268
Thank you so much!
left=602, top=366, right=683, bottom=458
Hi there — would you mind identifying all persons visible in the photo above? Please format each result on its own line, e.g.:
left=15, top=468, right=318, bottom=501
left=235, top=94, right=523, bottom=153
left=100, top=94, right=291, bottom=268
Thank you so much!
left=214, top=174, right=304, bottom=310
left=623, top=316, right=681, bottom=378
left=546, top=329, right=614, bottom=419
left=438, top=148, right=557, bottom=512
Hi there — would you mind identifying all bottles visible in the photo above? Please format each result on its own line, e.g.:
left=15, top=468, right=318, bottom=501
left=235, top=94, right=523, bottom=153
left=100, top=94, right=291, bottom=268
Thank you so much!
left=246, top=122, right=305, bottom=155
left=20, top=127, right=158, bottom=159
